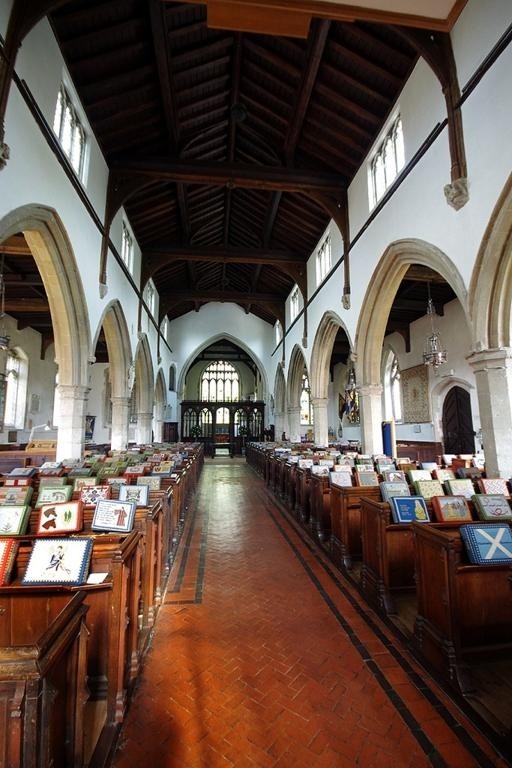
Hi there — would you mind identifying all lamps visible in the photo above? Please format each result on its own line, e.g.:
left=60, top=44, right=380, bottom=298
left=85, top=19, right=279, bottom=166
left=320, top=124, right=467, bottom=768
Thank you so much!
left=422, top=280, right=447, bottom=375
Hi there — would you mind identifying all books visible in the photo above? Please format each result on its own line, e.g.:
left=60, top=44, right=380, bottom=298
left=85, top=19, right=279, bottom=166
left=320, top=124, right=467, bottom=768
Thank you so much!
left=0, top=442, right=201, bottom=587
left=273, top=442, right=511, bottom=568
